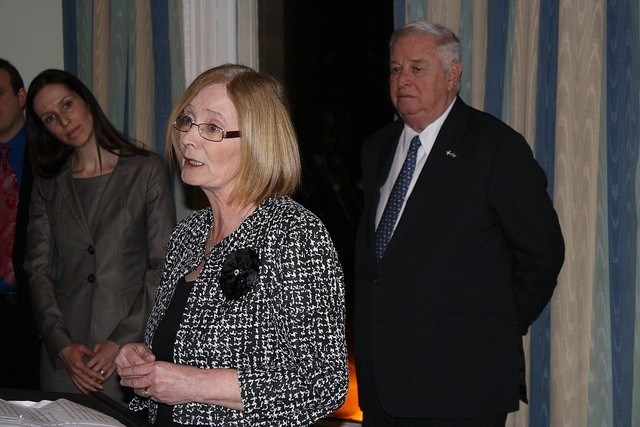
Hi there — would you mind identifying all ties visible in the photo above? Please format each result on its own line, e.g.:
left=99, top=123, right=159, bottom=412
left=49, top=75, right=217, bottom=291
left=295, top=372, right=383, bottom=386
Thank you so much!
left=375, top=136, right=421, bottom=263
left=0, top=144, right=19, bottom=290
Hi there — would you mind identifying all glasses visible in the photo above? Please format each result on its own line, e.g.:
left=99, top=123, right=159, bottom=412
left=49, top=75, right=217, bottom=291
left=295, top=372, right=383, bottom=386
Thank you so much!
left=173, top=114, right=241, bottom=143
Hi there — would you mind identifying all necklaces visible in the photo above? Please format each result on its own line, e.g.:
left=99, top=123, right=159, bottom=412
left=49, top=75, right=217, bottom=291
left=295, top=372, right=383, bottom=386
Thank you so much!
left=207, top=230, right=212, bottom=252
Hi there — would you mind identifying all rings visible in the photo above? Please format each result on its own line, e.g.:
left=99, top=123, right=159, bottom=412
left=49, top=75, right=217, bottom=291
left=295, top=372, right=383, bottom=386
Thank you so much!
left=101, top=370, right=106, bottom=376
left=143, top=387, right=150, bottom=397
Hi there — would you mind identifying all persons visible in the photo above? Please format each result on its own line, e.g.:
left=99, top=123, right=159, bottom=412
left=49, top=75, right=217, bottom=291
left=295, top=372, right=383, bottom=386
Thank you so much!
left=25, top=69, right=175, bottom=407
left=342, top=22, right=565, bottom=427
left=114, top=66, right=349, bottom=426
left=0, top=58, right=38, bottom=389
